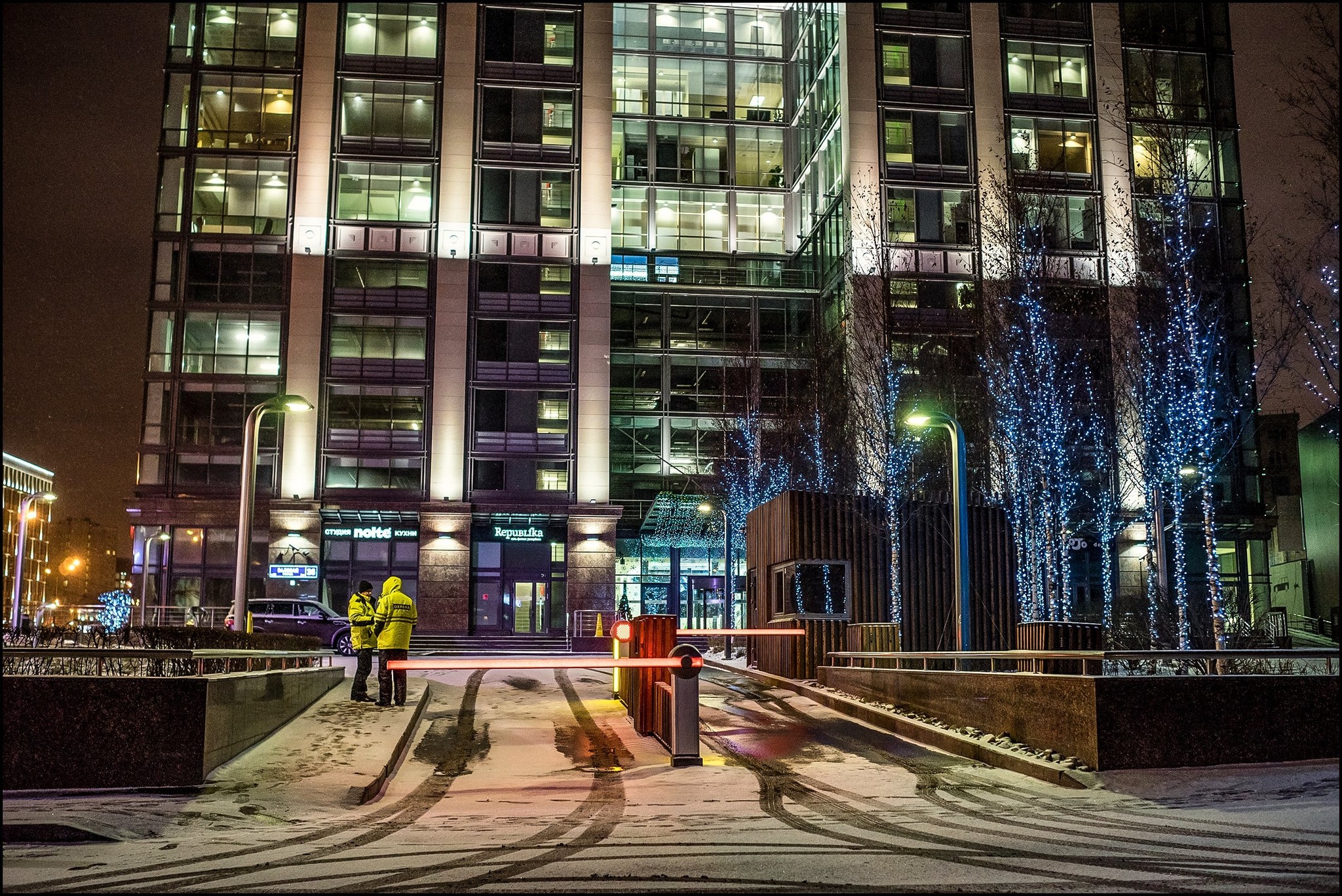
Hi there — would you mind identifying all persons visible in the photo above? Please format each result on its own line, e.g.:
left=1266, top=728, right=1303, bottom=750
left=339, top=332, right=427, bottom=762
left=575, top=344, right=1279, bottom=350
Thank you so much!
left=348, top=580, right=378, bottom=703
left=373, top=577, right=418, bottom=707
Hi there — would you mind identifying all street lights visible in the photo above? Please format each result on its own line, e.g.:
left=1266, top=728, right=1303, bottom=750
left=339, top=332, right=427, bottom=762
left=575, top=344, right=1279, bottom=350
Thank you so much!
left=13, top=491, right=58, bottom=636
left=233, top=394, right=314, bottom=633
left=1179, top=464, right=1228, bottom=650
left=904, top=408, right=976, bottom=672
left=697, top=503, right=734, bottom=660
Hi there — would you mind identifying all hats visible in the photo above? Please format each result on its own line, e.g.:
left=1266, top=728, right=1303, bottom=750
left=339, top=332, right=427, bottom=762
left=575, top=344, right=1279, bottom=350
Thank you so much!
left=359, top=580, right=373, bottom=593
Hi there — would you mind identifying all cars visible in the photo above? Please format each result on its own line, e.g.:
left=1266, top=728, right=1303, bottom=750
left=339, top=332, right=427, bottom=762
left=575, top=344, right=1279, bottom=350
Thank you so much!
left=225, top=597, right=360, bottom=657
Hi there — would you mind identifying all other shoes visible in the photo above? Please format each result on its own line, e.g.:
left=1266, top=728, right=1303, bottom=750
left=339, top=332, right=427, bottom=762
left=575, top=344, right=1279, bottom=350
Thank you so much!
left=395, top=701, right=404, bottom=706
left=350, top=693, right=376, bottom=702
left=375, top=699, right=390, bottom=707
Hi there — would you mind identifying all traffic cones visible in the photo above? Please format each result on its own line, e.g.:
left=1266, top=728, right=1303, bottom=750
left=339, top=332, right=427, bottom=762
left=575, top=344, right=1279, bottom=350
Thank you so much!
left=593, top=613, right=605, bottom=637
